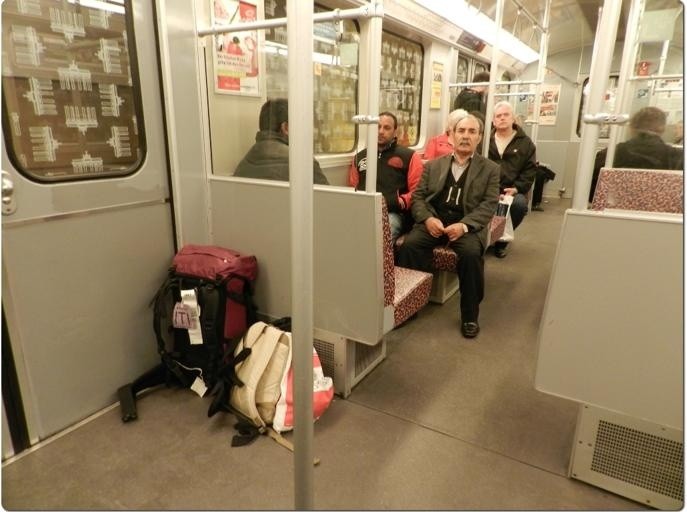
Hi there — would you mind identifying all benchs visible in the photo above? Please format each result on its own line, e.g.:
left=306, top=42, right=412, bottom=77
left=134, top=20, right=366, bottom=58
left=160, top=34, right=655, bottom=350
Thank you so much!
left=590, top=167, right=684, bottom=211
left=487, top=215, right=506, bottom=245
left=394, top=234, right=461, bottom=305
left=210, top=175, right=433, bottom=347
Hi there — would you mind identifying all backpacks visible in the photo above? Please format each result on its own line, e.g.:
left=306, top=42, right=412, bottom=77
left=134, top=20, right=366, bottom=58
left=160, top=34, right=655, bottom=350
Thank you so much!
left=117, top=245, right=259, bottom=422
left=226, top=318, right=292, bottom=446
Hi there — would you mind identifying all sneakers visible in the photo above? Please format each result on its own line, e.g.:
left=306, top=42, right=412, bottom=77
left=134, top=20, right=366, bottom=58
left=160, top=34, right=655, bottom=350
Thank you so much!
left=496, top=244, right=507, bottom=258
left=533, top=207, right=543, bottom=212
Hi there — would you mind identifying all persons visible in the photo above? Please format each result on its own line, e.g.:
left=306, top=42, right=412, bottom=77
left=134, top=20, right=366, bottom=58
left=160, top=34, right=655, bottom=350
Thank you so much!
left=227, top=35, right=245, bottom=55
left=423, top=108, right=470, bottom=162
left=392, top=115, right=502, bottom=339
left=231, top=97, right=331, bottom=186
left=671, top=119, right=684, bottom=150
left=588, top=105, right=684, bottom=209
left=349, top=110, right=424, bottom=250
left=452, top=72, right=557, bottom=259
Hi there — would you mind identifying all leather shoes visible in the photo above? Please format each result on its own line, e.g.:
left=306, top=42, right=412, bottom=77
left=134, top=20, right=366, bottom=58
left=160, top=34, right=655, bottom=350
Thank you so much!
left=461, top=321, right=479, bottom=337
